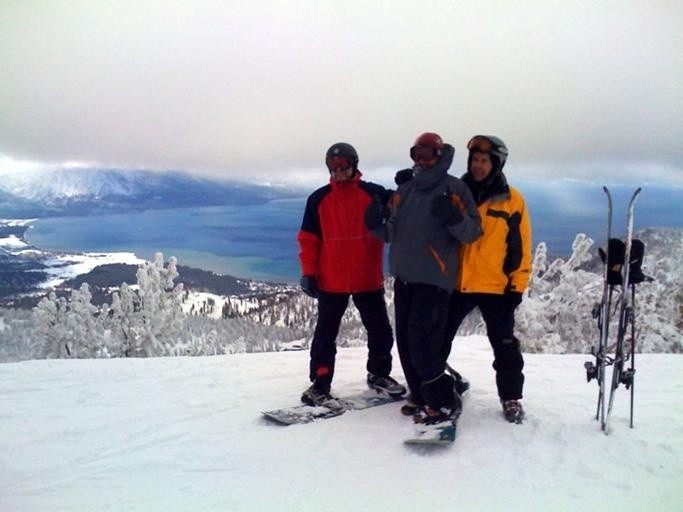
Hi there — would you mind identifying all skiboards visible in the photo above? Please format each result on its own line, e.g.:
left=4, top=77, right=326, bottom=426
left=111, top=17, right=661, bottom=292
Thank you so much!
left=400, top=379, right=524, bottom=424
left=585, top=184, right=644, bottom=437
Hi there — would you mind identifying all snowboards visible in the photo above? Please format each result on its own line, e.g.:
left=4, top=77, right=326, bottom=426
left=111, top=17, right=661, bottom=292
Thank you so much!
left=404, top=408, right=463, bottom=444
left=261, top=385, right=406, bottom=425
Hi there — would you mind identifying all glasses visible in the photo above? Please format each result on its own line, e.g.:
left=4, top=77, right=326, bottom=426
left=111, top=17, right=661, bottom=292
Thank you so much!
left=327, top=159, right=351, bottom=170
left=467, top=136, right=500, bottom=153
left=410, top=146, right=433, bottom=161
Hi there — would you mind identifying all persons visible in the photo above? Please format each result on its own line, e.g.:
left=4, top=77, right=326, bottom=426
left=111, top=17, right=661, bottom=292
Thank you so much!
left=362, top=132, right=485, bottom=433
left=297, top=142, right=398, bottom=408
left=398, top=133, right=533, bottom=425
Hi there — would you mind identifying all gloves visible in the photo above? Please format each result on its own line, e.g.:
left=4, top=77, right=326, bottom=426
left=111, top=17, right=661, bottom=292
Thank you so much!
left=364, top=202, right=390, bottom=229
left=394, top=170, right=413, bottom=185
left=432, top=193, right=466, bottom=223
left=302, top=276, right=320, bottom=297
left=598, top=239, right=644, bottom=284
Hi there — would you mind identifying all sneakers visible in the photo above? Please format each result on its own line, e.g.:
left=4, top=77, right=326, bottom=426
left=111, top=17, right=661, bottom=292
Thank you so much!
left=367, top=374, right=406, bottom=394
left=504, top=400, right=523, bottom=423
left=402, top=395, right=454, bottom=424
left=301, top=383, right=330, bottom=405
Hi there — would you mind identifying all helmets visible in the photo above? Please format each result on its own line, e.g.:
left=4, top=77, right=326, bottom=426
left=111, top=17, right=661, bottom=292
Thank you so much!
left=467, top=135, right=507, bottom=179
left=417, top=133, right=443, bottom=147
left=326, top=142, right=357, bottom=169
